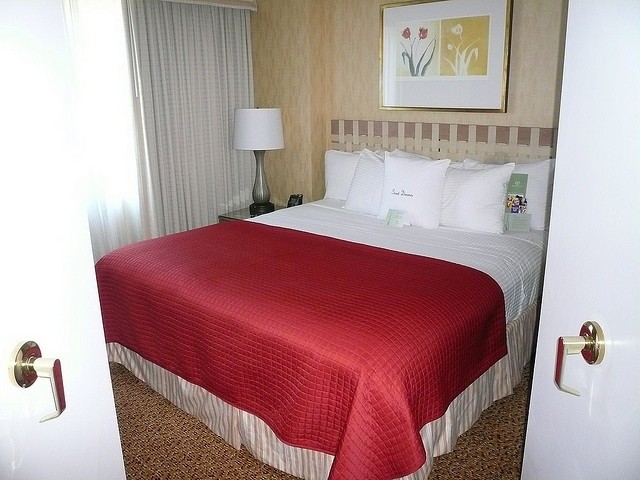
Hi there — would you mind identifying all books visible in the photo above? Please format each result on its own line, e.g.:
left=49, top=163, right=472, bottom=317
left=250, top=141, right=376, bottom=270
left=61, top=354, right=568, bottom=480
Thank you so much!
left=504, top=173, right=528, bottom=214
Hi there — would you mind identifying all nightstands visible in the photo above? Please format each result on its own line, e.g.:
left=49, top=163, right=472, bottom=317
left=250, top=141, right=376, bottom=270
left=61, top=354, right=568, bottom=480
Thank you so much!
left=218, top=205, right=287, bottom=222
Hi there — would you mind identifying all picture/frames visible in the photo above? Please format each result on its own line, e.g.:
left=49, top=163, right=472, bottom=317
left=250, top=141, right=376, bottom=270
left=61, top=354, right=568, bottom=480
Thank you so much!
left=378, top=0, right=514, bottom=113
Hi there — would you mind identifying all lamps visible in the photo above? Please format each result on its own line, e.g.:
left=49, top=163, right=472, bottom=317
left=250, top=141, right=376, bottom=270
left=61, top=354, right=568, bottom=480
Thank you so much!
left=233, top=108, right=285, bottom=216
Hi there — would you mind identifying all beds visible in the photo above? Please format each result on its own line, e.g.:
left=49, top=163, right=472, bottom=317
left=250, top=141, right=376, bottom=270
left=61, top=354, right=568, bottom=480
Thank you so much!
left=95, top=119, right=557, bottom=480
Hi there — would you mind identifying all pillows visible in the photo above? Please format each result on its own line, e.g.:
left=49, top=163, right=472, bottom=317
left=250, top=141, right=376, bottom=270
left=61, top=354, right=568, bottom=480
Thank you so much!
left=462, top=158, right=556, bottom=232
left=440, top=163, right=515, bottom=234
left=342, top=148, right=385, bottom=216
left=324, top=150, right=360, bottom=201
left=377, top=151, right=451, bottom=230
left=391, top=149, right=435, bottom=161
